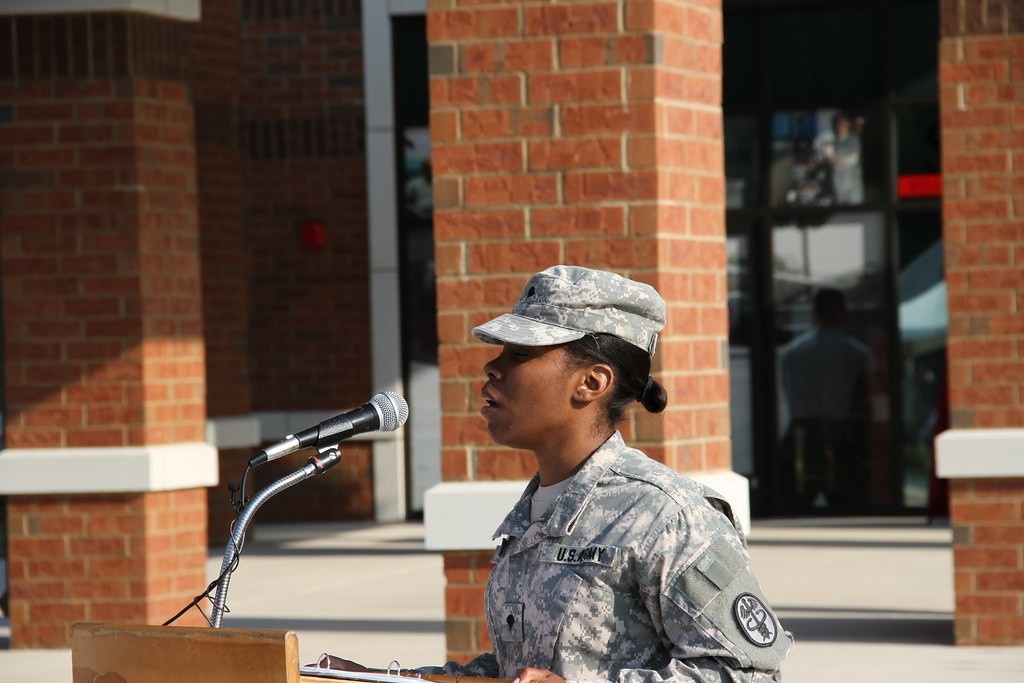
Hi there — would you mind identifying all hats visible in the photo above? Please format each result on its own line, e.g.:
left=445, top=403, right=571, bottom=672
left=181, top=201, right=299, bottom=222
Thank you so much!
left=473, top=264, right=666, bottom=357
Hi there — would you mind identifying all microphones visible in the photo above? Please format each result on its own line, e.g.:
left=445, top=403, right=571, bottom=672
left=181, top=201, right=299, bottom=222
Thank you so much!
left=247, top=391, right=410, bottom=468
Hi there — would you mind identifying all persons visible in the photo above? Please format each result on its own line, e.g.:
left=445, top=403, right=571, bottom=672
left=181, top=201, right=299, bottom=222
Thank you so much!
left=305, top=264, right=794, bottom=683
left=778, top=285, right=889, bottom=514
